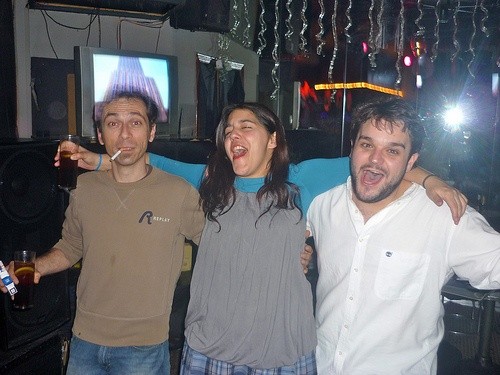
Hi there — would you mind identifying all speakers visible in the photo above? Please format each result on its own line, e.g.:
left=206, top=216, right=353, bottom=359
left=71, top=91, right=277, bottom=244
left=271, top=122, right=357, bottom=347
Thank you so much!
left=0, top=333, right=67, bottom=375
left=254, top=0, right=304, bottom=55
left=0, top=137, right=76, bottom=352
left=31, top=56, right=74, bottom=139
left=170, top=0, right=232, bottom=34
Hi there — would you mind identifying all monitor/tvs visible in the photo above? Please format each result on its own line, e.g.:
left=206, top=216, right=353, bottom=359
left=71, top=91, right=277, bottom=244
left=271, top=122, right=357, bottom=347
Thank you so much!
left=75, top=46, right=179, bottom=136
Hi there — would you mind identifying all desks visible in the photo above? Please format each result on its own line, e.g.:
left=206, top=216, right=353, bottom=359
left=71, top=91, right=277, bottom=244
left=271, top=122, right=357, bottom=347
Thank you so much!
left=442, top=275, right=500, bottom=375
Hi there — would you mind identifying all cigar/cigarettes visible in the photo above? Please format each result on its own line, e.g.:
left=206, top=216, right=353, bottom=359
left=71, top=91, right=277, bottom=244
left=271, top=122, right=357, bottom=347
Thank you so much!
left=110, top=148, right=123, bottom=161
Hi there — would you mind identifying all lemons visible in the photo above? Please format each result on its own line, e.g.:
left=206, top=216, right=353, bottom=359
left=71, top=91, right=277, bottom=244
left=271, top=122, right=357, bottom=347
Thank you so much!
left=15, top=267, right=35, bottom=274
left=63, top=154, right=72, bottom=158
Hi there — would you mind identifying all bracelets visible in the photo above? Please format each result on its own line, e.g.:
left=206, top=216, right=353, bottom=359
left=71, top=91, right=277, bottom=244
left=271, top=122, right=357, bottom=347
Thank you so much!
left=96, top=153, right=102, bottom=170
left=422, top=174, right=441, bottom=187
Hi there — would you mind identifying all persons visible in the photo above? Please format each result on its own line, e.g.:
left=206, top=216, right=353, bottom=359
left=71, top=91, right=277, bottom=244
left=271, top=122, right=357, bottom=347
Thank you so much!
left=1, top=92, right=314, bottom=374
left=55, top=101, right=468, bottom=374
left=306, top=96, right=500, bottom=375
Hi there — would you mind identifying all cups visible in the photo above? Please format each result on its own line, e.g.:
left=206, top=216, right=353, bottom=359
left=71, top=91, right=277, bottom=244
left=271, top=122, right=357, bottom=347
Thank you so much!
left=57, top=134, right=81, bottom=190
left=13, top=250, right=36, bottom=310
